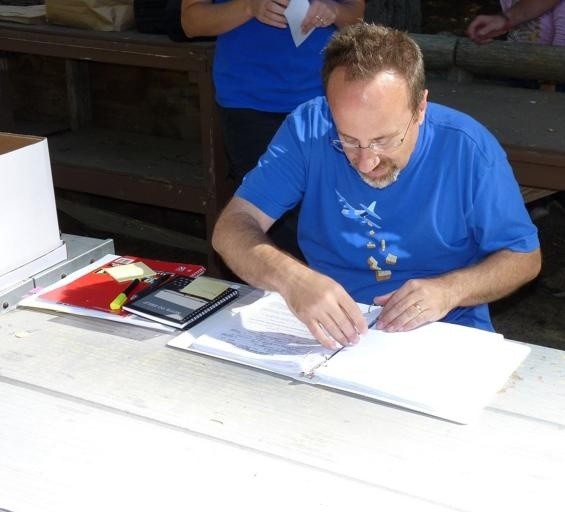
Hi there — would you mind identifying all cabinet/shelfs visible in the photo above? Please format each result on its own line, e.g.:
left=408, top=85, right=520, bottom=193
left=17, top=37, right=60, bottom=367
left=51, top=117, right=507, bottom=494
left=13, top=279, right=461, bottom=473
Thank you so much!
left=0, top=20, right=233, bottom=277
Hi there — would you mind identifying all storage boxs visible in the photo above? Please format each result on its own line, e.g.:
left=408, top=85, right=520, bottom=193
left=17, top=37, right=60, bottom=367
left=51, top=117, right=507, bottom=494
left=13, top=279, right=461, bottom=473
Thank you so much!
left=0, top=132, right=67, bottom=293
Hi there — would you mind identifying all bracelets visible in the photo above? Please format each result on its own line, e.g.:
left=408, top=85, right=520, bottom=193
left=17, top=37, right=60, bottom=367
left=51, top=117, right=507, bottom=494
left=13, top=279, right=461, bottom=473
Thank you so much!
left=501, top=12, right=514, bottom=29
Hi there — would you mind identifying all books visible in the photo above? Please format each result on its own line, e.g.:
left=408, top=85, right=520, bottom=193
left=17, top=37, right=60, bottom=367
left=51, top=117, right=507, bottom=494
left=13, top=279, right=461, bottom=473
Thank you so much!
left=39, top=256, right=206, bottom=316
left=122, top=274, right=241, bottom=330
left=166, top=290, right=532, bottom=424
left=1, top=4, right=46, bottom=25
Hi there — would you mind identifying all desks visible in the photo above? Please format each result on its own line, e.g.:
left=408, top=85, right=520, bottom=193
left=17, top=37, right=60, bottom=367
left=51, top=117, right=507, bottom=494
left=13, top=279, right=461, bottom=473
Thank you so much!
left=0, top=253, right=565, bottom=512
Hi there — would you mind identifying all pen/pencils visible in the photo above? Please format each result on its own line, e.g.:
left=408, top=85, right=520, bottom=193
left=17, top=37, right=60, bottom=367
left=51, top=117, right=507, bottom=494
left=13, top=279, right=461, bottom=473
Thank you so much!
left=129, top=274, right=171, bottom=302
left=109, top=278, right=140, bottom=310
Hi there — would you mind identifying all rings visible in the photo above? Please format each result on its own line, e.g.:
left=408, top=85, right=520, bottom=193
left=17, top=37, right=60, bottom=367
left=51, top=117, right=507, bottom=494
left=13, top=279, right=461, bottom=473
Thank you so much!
left=413, top=303, right=424, bottom=312
left=316, top=15, right=320, bottom=20
left=320, top=18, right=324, bottom=23
left=324, top=20, right=329, bottom=24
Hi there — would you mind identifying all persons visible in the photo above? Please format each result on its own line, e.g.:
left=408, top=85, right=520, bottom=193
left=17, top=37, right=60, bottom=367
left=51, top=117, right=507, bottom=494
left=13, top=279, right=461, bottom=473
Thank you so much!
left=210, top=19, right=542, bottom=351
left=181, top=0, right=367, bottom=279
left=465, top=0, right=565, bottom=93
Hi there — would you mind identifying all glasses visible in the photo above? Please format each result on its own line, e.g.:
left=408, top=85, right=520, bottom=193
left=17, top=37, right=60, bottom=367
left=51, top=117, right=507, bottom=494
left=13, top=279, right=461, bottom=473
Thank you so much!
left=328, top=105, right=418, bottom=154
